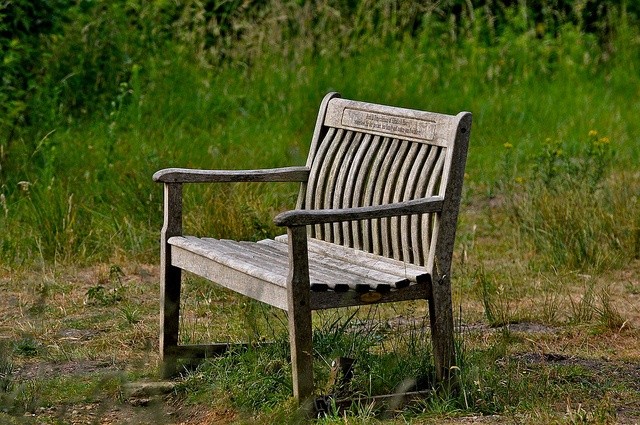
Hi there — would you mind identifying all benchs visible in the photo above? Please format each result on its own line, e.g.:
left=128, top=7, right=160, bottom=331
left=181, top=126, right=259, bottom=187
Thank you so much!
left=151, top=91, right=474, bottom=407
